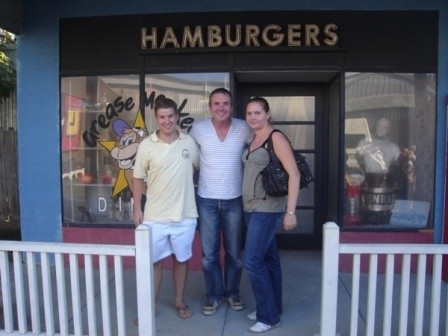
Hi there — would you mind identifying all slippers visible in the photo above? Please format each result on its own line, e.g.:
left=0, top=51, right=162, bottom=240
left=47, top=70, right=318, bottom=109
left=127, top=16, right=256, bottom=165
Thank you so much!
left=173, top=304, right=191, bottom=319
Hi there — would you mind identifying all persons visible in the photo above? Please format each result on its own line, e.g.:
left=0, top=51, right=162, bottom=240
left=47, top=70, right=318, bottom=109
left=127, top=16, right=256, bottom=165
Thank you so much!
left=132, top=98, right=201, bottom=326
left=240, top=96, right=300, bottom=333
left=357, top=118, right=399, bottom=188
left=188, top=88, right=256, bottom=316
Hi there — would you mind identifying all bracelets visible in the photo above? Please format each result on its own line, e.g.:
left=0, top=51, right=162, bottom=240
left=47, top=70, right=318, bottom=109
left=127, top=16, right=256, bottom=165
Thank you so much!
left=286, top=211, right=296, bottom=216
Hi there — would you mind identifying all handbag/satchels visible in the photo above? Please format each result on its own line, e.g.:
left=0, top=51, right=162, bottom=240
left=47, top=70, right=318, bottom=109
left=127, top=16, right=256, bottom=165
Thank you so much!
left=261, top=130, right=314, bottom=197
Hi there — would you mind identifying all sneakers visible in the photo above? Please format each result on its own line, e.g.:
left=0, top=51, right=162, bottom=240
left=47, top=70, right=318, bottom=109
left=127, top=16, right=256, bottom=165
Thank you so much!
left=202, top=298, right=222, bottom=316
left=228, top=295, right=243, bottom=311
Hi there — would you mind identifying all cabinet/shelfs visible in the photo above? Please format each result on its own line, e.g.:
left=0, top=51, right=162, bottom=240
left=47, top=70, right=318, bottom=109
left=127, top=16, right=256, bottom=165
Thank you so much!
left=68, top=109, right=133, bottom=223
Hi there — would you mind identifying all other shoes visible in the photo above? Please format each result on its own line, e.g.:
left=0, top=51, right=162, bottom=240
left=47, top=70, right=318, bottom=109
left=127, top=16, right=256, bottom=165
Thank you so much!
left=250, top=321, right=280, bottom=332
left=248, top=310, right=258, bottom=320
